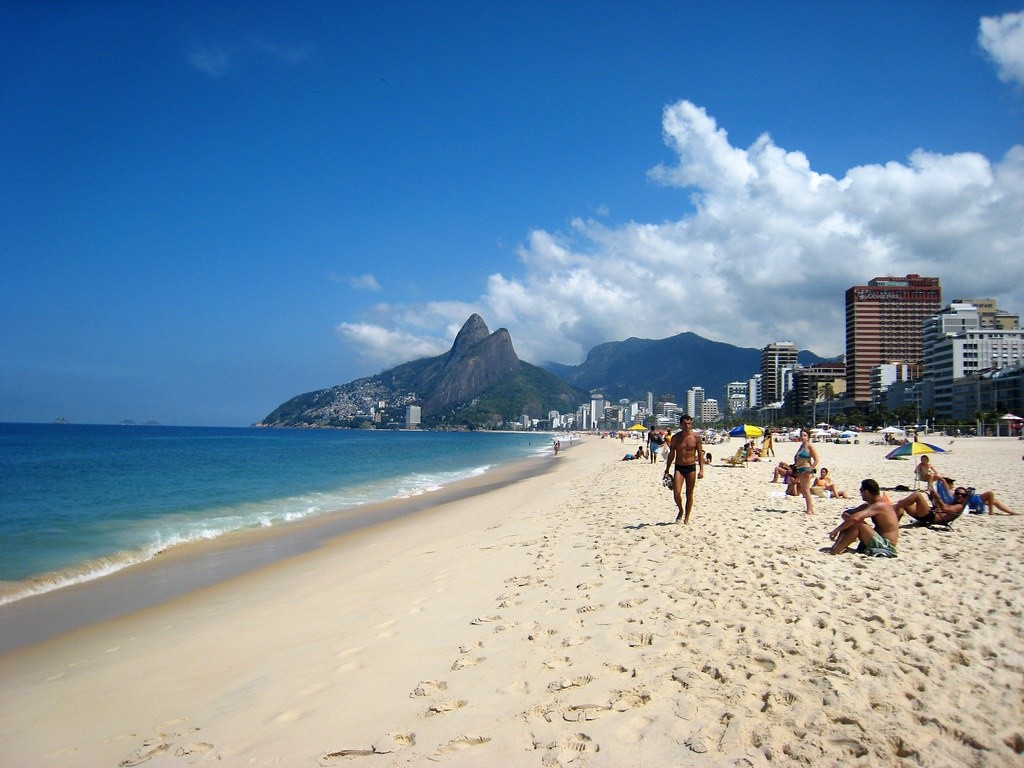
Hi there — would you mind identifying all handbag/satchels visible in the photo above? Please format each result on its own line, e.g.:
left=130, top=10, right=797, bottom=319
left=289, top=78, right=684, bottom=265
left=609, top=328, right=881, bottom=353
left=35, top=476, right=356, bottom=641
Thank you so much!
left=654, top=433, right=665, bottom=445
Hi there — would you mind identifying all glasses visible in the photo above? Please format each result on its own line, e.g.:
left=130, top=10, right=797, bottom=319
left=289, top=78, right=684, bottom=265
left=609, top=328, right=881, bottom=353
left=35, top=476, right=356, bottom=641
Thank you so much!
left=956, top=492, right=967, bottom=499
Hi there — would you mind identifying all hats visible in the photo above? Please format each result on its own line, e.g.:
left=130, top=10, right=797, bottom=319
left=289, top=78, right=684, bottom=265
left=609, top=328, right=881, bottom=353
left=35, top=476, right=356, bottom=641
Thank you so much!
left=667, top=427, right=671, bottom=431
left=944, top=478, right=956, bottom=484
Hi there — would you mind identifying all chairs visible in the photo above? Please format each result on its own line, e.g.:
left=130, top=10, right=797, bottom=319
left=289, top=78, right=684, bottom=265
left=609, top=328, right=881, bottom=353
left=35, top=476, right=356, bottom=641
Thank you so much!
left=925, top=487, right=976, bottom=532
left=721, top=447, right=752, bottom=468
left=914, top=471, right=929, bottom=491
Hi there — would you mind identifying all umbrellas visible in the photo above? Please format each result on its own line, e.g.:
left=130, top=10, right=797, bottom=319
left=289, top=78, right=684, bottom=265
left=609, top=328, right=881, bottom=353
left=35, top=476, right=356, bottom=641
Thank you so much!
left=728, top=424, right=766, bottom=444
left=998, top=412, right=1023, bottom=436
left=788, top=422, right=860, bottom=441
left=877, top=426, right=905, bottom=434
left=884, top=441, right=949, bottom=479
left=628, top=423, right=648, bottom=433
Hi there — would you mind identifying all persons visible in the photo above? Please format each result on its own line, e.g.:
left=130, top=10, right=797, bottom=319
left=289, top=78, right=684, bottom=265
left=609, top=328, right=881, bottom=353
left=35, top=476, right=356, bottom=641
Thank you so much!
left=824, top=479, right=898, bottom=558
left=795, top=429, right=819, bottom=515
left=667, top=414, right=704, bottom=526
left=954, top=423, right=1024, bottom=437
left=883, top=455, right=1024, bottom=525
left=553, top=441, right=561, bottom=456
left=769, top=462, right=817, bottom=498
left=721, top=429, right=775, bottom=464
left=622, top=446, right=646, bottom=461
left=620, top=431, right=645, bottom=443
left=812, top=468, right=854, bottom=499
left=695, top=450, right=712, bottom=465
left=647, top=426, right=672, bottom=464
left=885, top=433, right=893, bottom=444
